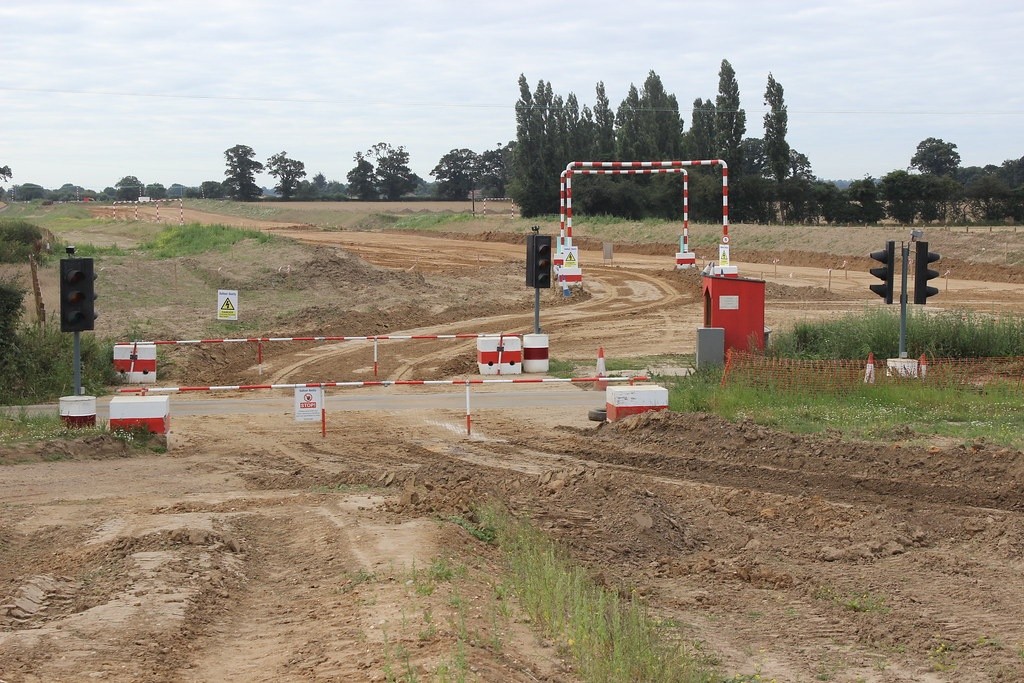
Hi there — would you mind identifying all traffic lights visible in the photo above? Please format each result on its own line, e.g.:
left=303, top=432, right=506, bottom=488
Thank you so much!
left=912, top=241, right=940, bottom=303
left=82, top=257, right=99, bottom=333
left=869, top=240, right=896, bottom=305
left=534, top=235, right=551, bottom=289
left=60, top=257, right=88, bottom=332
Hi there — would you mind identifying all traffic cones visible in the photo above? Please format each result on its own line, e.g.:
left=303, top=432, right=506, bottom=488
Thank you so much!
left=863, top=352, right=876, bottom=386
left=918, top=353, right=929, bottom=383
left=591, top=347, right=612, bottom=392
